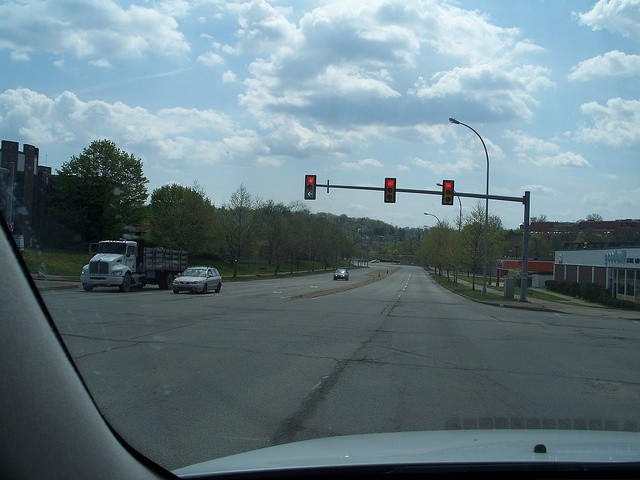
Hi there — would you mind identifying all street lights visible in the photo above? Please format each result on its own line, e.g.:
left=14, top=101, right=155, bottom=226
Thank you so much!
left=436, top=182, right=463, bottom=234
left=449, top=117, right=489, bottom=298
left=424, top=212, right=442, bottom=230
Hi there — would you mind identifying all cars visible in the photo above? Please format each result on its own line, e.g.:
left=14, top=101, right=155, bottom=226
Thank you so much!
left=334, top=268, right=349, bottom=281
left=172, top=267, right=222, bottom=293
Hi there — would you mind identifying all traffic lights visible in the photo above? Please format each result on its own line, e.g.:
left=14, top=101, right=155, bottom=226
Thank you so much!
left=384, top=178, right=396, bottom=204
left=442, top=180, right=454, bottom=205
left=304, top=175, right=317, bottom=200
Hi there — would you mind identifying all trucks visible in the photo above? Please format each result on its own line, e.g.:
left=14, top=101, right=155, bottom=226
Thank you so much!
left=80, top=240, right=189, bottom=292
